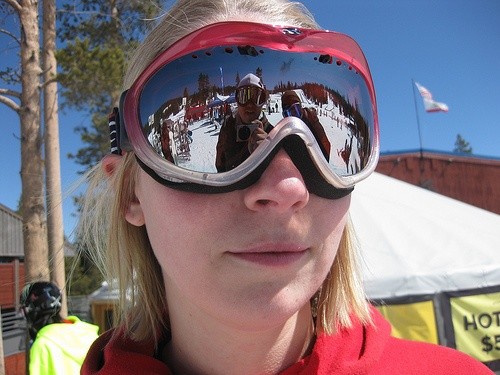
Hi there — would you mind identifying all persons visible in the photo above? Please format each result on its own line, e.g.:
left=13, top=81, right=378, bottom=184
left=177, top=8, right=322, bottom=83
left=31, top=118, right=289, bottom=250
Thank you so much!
left=19, top=281, right=100, bottom=375
left=78, top=0, right=497, bottom=375
left=215, top=73, right=274, bottom=172
left=282, top=89, right=330, bottom=164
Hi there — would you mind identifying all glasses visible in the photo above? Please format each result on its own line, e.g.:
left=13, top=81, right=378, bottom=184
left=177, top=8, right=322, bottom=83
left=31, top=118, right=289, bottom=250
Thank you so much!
left=108, top=21, right=380, bottom=199
left=236, top=85, right=267, bottom=108
left=282, top=103, right=304, bottom=119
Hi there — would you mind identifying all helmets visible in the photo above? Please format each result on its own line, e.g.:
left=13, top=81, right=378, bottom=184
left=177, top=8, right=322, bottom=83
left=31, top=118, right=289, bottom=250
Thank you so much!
left=20, top=280, right=63, bottom=318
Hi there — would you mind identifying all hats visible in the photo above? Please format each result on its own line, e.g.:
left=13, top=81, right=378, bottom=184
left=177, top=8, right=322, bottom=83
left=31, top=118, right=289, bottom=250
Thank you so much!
left=236, top=74, right=264, bottom=89
left=280, top=91, right=300, bottom=104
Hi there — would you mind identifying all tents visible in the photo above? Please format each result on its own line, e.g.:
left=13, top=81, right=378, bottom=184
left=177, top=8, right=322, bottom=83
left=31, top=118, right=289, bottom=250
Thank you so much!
left=93, top=172, right=500, bottom=369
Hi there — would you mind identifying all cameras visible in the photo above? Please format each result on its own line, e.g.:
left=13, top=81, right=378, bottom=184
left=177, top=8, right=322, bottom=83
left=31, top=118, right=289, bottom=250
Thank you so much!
left=236, top=123, right=259, bottom=142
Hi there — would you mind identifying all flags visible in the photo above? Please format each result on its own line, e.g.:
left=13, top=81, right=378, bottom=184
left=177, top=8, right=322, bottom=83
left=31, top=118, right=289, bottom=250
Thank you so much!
left=414, top=82, right=449, bottom=113
